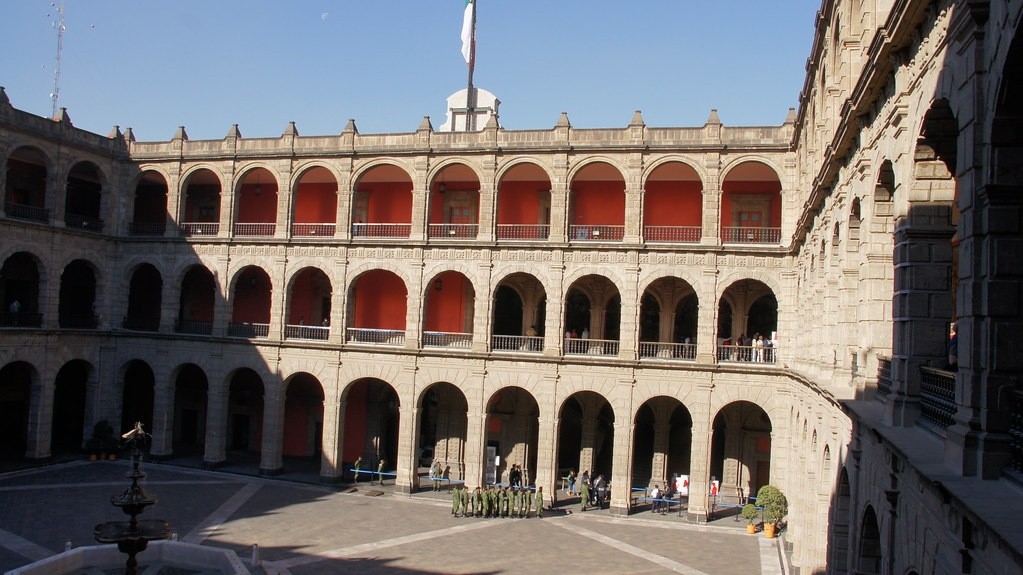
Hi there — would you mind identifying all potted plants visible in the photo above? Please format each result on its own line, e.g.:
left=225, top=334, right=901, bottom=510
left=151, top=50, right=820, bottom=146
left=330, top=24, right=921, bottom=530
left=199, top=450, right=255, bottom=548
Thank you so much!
left=108, top=439, right=117, bottom=460
left=93, top=420, right=114, bottom=461
left=87, top=439, right=98, bottom=461
left=763, top=498, right=781, bottom=537
left=742, top=504, right=758, bottom=534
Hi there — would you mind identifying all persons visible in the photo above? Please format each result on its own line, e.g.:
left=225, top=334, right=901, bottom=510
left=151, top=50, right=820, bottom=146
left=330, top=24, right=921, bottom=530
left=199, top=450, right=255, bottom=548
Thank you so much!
left=683, top=332, right=774, bottom=363
left=947, top=320, right=958, bottom=372
left=451, top=484, right=543, bottom=519
left=295, top=315, right=305, bottom=339
left=651, top=485, right=660, bottom=513
left=321, top=318, right=330, bottom=340
left=354, top=456, right=363, bottom=485
left=661, top=485, right=672, bottom=514
left=566, top=470, right=607, bottom=511
left=430, top=461, right=443, bottom=492
left=509, top=464, right=522, bottom=487
left=377, top=459, right=385, bottom=486
left=518, top=325, right=591, bottom=355
